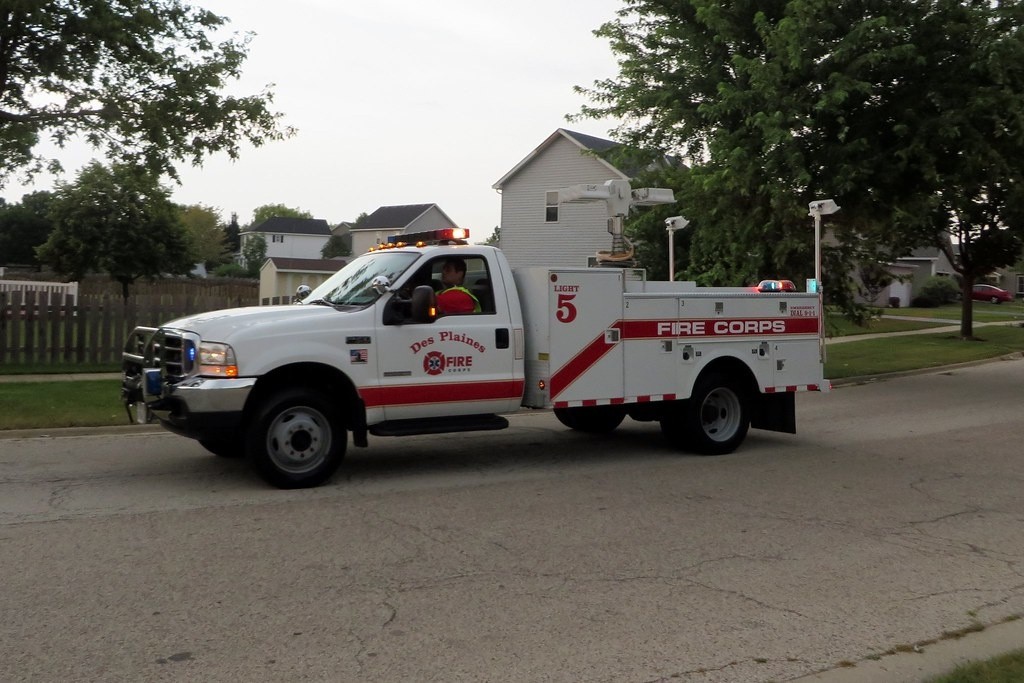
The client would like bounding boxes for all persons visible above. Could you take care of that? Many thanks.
[432,258,481,315]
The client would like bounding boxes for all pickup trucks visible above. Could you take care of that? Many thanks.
[121,180,842,490]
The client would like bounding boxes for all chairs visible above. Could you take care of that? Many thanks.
[471,278,490,296]
[432,278,444,291]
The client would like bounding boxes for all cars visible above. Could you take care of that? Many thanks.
[957,283,1012,305]
